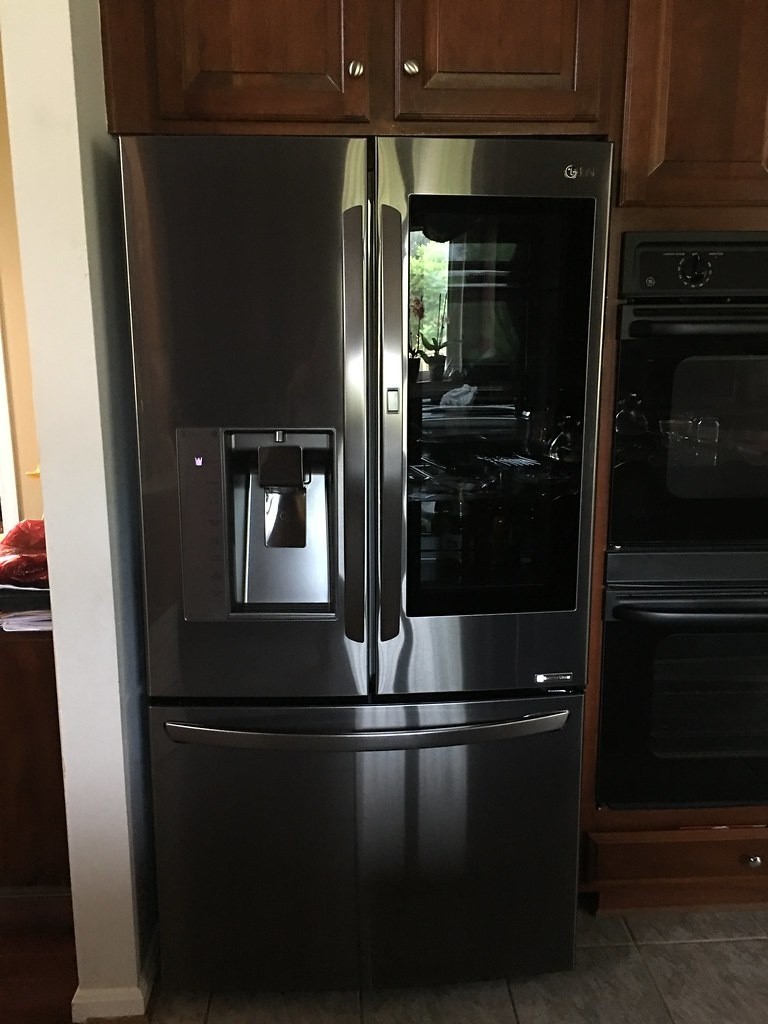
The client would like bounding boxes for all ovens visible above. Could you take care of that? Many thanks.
[593,226,768,810]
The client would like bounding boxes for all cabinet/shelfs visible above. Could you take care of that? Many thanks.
[99,1,611,144]
[579,0,768,916]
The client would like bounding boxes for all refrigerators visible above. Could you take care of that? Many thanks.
[117,132,615,999]
